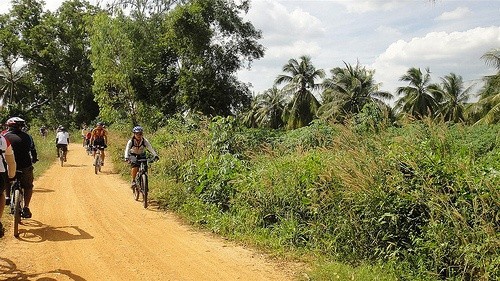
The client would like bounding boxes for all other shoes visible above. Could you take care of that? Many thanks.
[23,207,32,218]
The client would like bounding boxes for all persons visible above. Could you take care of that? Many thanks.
[82,126,89,147]
[55,125,69,162]
[3,117,37,217]
[124,126,159,187]
[0,134,17,232]
[86,128,94,148]
[89,122,108,166]
[40,126,46,136]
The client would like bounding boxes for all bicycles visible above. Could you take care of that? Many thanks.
[124,157,155,208]
[10,169,28,237]
[89,145,105,173]
[58,146,66,166]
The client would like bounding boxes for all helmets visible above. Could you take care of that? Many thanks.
[5,117,26,127]
[132,126,143,133]
[96,122,103,126]
